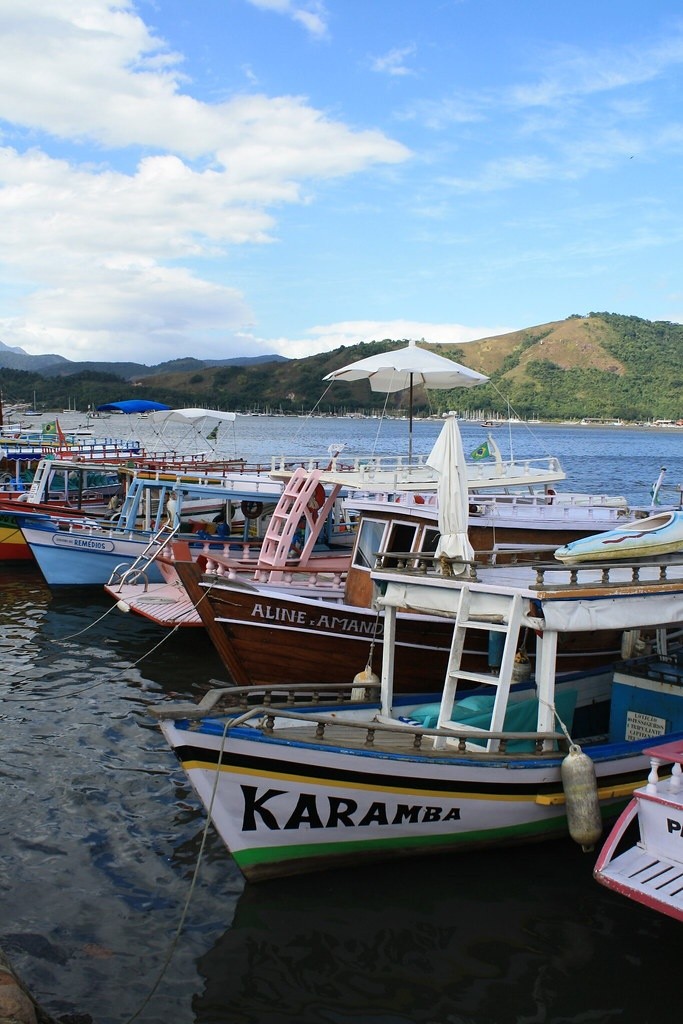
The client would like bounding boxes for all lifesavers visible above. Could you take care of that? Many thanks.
[221,504,236,519]
[240,500,264,519]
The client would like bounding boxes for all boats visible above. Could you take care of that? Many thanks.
[0,405,681,921]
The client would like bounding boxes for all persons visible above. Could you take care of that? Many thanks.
[167,491,181,533]
[288,480,326,558]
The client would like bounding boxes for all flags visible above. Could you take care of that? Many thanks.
[41,420,57,435]
[470,442,490,462]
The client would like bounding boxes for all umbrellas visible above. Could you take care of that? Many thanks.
[426,410,475,578]
[322,339,492,477]
